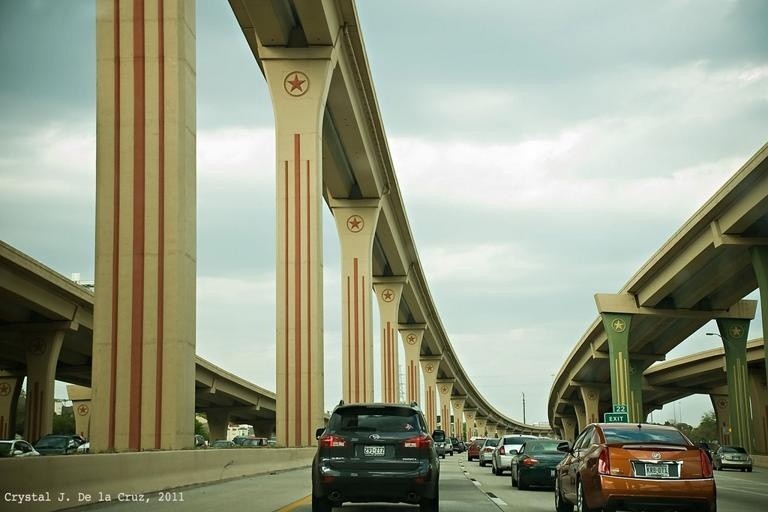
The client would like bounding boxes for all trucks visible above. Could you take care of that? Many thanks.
[241,437,269,447]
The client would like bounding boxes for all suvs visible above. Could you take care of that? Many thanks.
[694,439,719,464]
[311,398,445,510]
[34,434,86,456]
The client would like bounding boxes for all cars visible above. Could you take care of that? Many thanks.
[479,438,502,466]
[77,439,89,454]
[711,444,753,473]
[452,438,462,453]
[211,439,235,447]
[492,434,538,475]
[267,436,276,444]
[0,439,40,457]
[194,434,206,448]
[459,437,473,451]
[443,437,454,458]
[434,440,445,459]
[554,421,717,510]
[468,439,486,461]
[510,439,573,489]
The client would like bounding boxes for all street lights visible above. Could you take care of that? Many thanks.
[706,332,721,341]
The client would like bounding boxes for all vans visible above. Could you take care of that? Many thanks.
[232,435,255,447]
